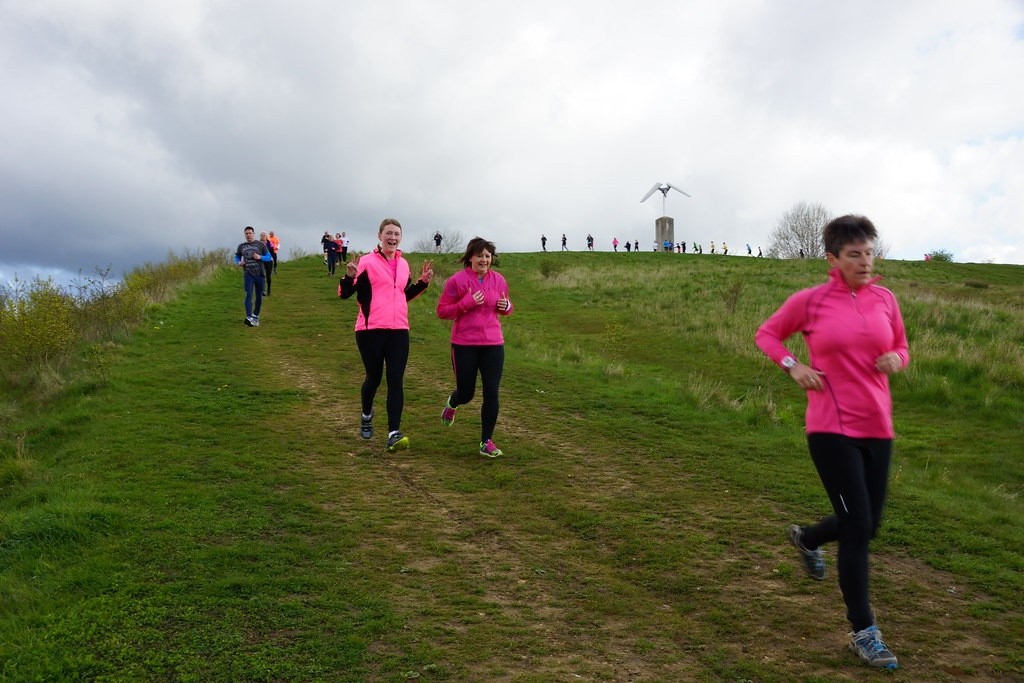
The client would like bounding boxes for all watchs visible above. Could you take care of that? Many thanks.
[782,356,799,372]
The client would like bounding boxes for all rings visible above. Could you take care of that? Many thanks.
[476,296,478,298]
[809,379,814,381]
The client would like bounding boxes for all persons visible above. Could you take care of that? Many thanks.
[561,233,569,251]
[799,249,804,258]
[924,254,930,260]
[587,234,593,250]
[434,231,442,254]
[234,226,271,326]
[746,244,763,257]
[756,215,909,668]
[268,231,279,274]
[260,231,274,296]
[693,241,702,253]
[711,241,728,254]
[652,240,686,253]
[613,238,638,252]
[338,219,434,453]
[321,232,350,276]
[436,238,514,457]
[541,234,547,251]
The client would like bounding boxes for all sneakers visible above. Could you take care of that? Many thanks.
[441,396,458,427]
[479,439,503,459]
[848,624,898,670]
[360,408,373,439]
[323,261,327,265]
[243,313,259,327]
[787,524,828,580]
[262,290,266,296]
[267,293,272,296]
[384,432,410,455]
